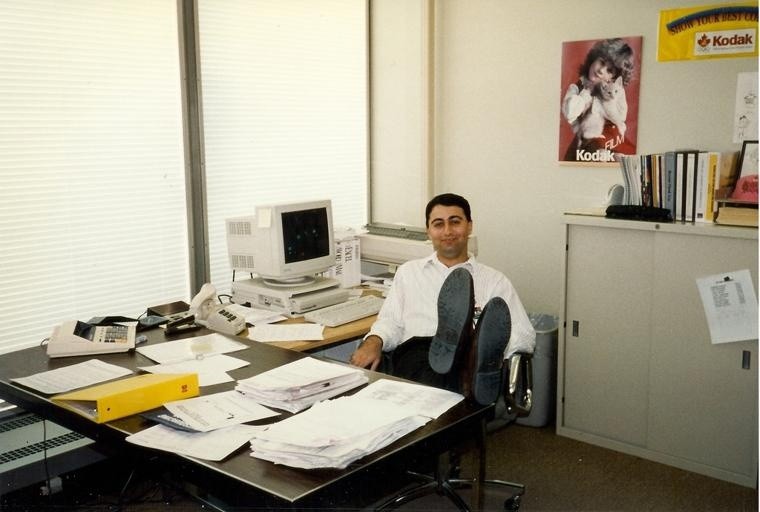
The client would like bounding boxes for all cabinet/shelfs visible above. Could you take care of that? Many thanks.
[557,209,759,490]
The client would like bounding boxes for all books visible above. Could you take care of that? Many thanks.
[234,356,431,469]
[139,410,199,434]
[613,139,758,233]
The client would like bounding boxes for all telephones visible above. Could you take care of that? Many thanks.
[189,283,246,335]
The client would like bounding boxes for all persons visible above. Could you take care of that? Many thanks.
[560,37,635,163]
[350,192,537,410]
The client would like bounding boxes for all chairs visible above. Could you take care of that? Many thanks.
[375,351,536,511]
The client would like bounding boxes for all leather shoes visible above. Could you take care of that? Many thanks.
[427,266,477,374]
[468,296,510,408]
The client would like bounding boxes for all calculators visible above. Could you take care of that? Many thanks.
[46,320,136,358]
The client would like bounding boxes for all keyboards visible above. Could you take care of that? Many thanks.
[303,294,384,328]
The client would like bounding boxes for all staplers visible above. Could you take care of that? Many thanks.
[164,315,202,335]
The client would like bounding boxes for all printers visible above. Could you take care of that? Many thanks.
[356,222,478,281]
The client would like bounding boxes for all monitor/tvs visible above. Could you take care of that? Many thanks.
[224,199,335,288]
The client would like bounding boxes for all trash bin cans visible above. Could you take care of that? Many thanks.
[515,311,558,427]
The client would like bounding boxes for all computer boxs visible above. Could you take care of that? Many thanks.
[231,275,349,318]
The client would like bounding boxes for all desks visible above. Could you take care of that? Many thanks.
[0,280,497,512]
